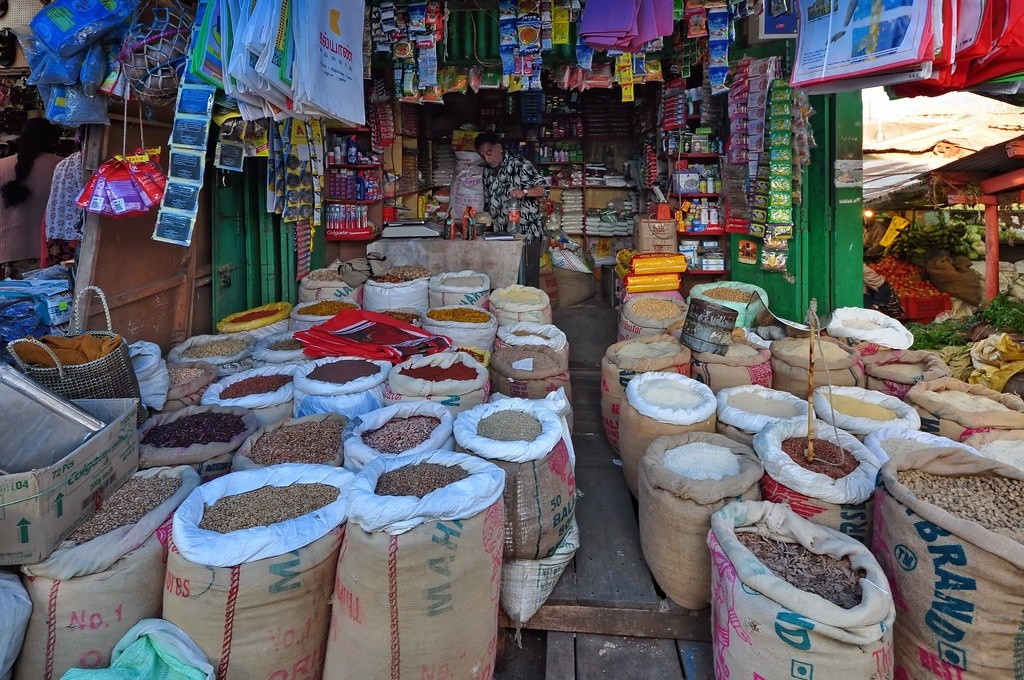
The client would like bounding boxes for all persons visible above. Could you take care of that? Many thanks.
[0,118,65,280]
[474,130,550,289]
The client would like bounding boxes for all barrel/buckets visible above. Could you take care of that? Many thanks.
[680,298,739,355]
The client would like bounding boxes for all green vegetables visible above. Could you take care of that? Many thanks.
[902,292,1024,351]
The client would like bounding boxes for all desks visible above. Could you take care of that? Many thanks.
[367,234,523,290]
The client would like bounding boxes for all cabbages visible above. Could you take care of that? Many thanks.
[952,225,1016,261]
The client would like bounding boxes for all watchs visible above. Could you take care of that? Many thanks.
[522,188,528,198]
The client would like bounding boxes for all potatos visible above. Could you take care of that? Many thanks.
[123,40,190,89]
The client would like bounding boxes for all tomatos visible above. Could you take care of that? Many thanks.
[869,255,942,297]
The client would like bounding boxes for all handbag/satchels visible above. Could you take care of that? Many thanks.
[291,305,453,362]
[7,285,148,430]
[16,0,367,127]
[789,0,1024,101]
[45,150,85,240]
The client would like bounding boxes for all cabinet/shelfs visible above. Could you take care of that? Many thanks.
[325,89,732,274]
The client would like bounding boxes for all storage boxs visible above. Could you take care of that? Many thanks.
[601,264,624,308]
[452,129,485,151]
[634,217,679,254]
[0,398,141,567]
[672,171,700,194]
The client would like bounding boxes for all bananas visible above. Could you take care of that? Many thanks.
[890,223,968,267]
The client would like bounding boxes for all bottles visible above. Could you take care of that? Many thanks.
[710,209,717,224]
[507,199,519,233]
[553,143,584,163]
[699,175,722,193]
[701,209,708,224]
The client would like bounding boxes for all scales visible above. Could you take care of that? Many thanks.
[381,217,445,240]
